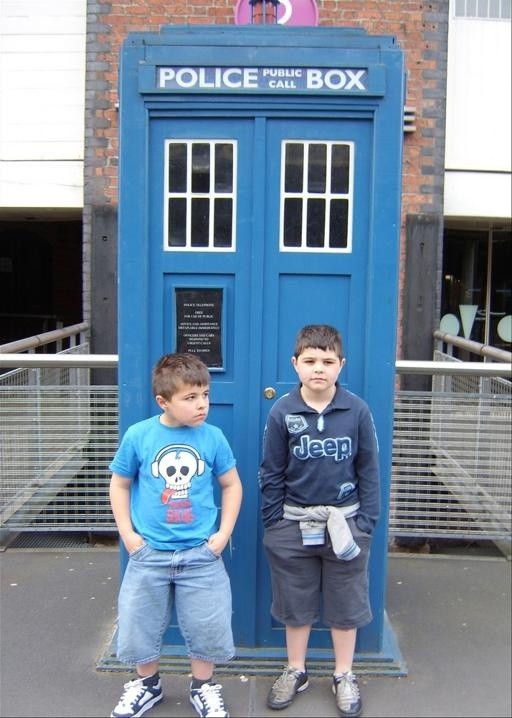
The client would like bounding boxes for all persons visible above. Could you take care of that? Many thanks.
[257,323,380,718]
[108,353,243,718]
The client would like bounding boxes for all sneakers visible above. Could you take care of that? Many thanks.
[267,663,309,709]
[110,674,164,718]
[189,679,229,718]
[331,671,362,718]
